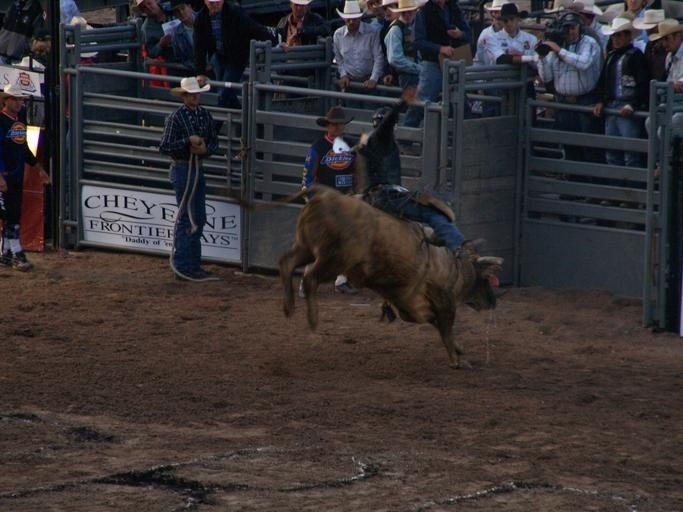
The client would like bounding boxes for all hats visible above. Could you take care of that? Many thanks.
[316,106,353,126]
[65,15,98,58]
[0,85,30,103]
[132,0,202,12]
[335,0,422,19]
[544,1,683,42]
[484,0,528,20]
[172,77,210,97]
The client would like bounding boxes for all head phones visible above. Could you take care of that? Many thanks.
[559,13,586,34]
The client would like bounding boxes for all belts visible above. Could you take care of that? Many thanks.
[171,159,201,168]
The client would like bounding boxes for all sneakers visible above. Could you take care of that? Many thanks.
[0,249,32,272]
[335,282,358,295]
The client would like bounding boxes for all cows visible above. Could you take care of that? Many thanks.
[227,183,505,369]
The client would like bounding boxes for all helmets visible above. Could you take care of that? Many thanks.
[371,107,398,129]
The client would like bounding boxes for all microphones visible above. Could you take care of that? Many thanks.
[572,34,585,44]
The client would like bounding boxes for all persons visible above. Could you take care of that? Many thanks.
[295,106,357,298]
[0,84,51,272]
[1,2,682,232]
[159,77,214,282]
[354,85,487,258]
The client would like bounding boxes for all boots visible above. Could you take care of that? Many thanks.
[395,105,424,156]
